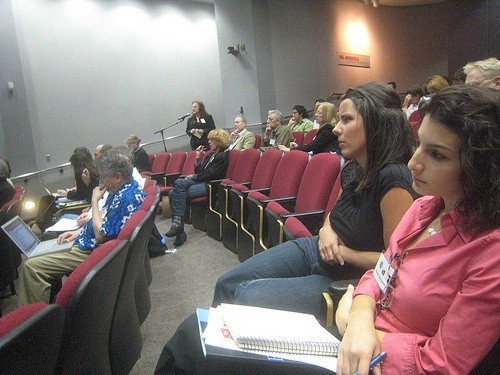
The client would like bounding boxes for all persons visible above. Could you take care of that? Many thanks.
[259,110,294,152]
[154,85,500,375]
[186,101,216,151]
[212,80,417,320]
[224,115,256,152]
[287,105,314,132]
[314,99,327,129]
[165,129,231,246]
[386,57,500,120]
[278,102,341,155]
[19,135,149,307]
[0,159,16,210]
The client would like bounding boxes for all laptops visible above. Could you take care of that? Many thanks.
[42,184,68,198]
[1,215,76,259]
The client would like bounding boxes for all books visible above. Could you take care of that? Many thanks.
[196,302,343,373]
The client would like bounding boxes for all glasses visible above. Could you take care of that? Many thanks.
[341,87,364,101]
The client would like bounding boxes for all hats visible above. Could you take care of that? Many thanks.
[124,134,142,143]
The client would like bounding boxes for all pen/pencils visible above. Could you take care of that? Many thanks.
[354,351,387,375]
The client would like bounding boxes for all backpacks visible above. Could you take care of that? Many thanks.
[147,224,167,259]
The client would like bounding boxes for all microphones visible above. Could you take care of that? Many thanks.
[178,112,190,120]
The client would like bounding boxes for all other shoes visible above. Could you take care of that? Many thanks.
[173,232,187,247]
[165,222,184,237]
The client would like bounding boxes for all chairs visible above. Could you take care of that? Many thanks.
[303,129,318,145]
[408,110,423,143]
[0,185,26,296]
[254,136,261,149]
[0,302,65,375]
[54,239,129,375]
[107,148,347,375]
[292,132,305,144]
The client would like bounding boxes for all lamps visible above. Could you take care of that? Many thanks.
[228,43,246,53]
[365,0,379,7]
[8,82,13,90]
